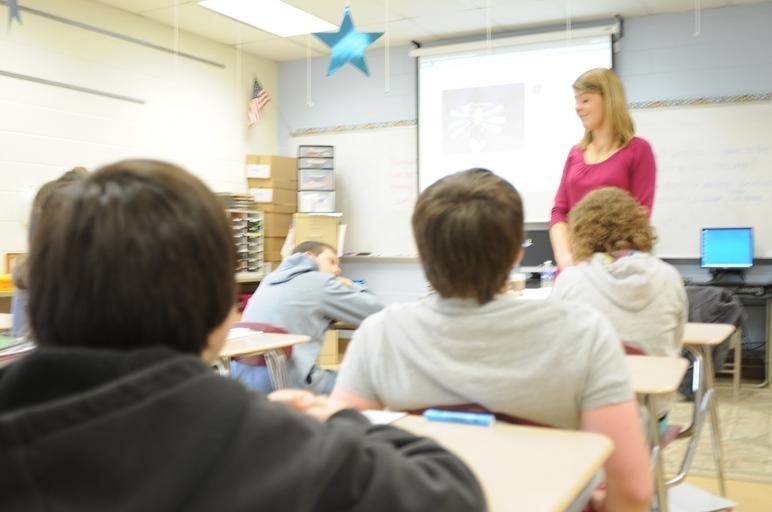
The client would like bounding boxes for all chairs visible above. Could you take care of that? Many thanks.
[679,286,742,401]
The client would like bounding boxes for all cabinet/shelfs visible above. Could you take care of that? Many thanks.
[292,212,343,266]
[224,210,264,271]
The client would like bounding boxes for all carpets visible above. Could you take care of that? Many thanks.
[662,378,772,485]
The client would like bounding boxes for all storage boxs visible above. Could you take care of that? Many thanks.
[298,158,334,169]
[298,144,334,158]
[298,170,335,191]
[263,261,282,276]
[297,191,335,213]
[247,187,299,213]
[254,210,293,237]
[263,237,287,261]
[247,153,298,190]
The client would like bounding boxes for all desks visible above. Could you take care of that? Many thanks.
[623,354,690,512]
[682,281,772,388]
[218,327,310,390]
[683,322,737,499]
[317,322,359,372]
[267,389,616,512]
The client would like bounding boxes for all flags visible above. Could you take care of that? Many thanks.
[247,78,271,130]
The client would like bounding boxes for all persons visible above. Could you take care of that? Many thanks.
[329,165,658,512]
[228,239,387,401]
[2,157,487,512]
[551,184,689,446]
[9,253,29,340]
[547,67,657,278]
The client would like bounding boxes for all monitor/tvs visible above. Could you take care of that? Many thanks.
[701,227,753,286]
[517,230,558,289]
